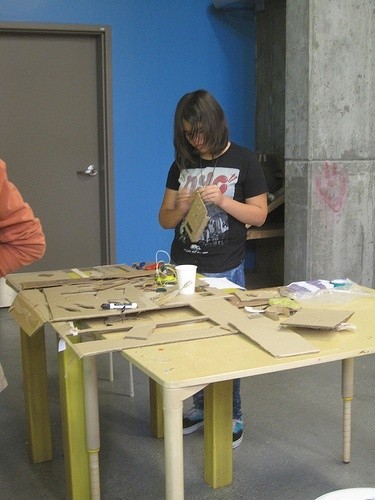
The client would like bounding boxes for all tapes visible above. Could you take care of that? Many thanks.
[330,278,347,287]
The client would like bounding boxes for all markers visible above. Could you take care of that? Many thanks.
[101,302,139,310]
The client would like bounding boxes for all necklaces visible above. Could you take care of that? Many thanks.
[199,153,216,188]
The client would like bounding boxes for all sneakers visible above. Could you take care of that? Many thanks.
[232,414,244,448]
[183,407,204,435]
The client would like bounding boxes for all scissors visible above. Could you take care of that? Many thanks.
[131,261,145,270]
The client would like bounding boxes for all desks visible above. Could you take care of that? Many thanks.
[5,269,375,500]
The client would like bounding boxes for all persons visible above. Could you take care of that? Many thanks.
[159,89,268,448]
[0,158,46,278]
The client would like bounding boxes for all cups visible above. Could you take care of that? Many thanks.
[175,265,198,294]
